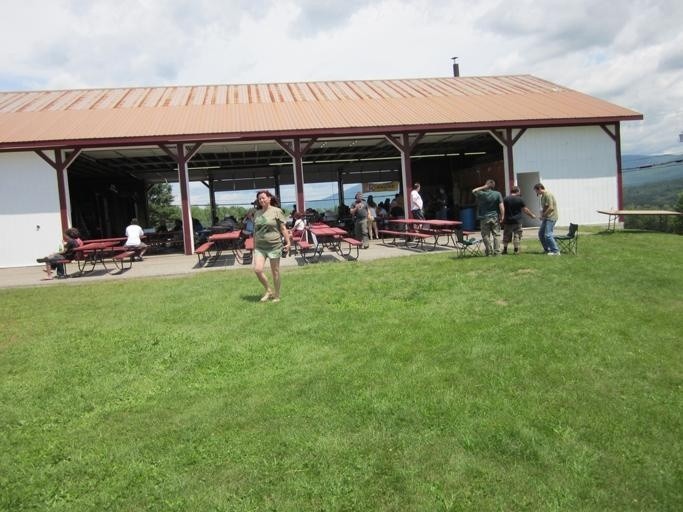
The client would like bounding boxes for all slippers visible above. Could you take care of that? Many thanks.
[261,295,272,302]
[273,298,280,302]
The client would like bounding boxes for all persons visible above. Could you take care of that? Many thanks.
[236,207,254,247]
[534,183,560,256]
[125,218,149,261]
[43,227,81,274]
[342,190,405,249]
[436,187,448,219]
[284,212,304,247]
[156,221,169,248]
[192,217,204,244]
[292,204,296,228]
[57,228,83,277]
[306,208,323,222]
[501,185,536,254]
[173,221,184,246]
[249,191,291,302]
[411,183,425,232]
[471,180,505,255]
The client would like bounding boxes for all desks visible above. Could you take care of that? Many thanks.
[83,234,147,262]
[597,208,682,234]
[208,229,243,267]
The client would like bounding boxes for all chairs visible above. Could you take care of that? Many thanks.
[454,226,483,258]
[553,223,579,256]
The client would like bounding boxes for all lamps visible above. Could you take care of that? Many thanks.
[172,165,222,171]
[268,152,488,166]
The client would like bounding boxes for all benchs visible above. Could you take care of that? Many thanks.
[37,242,140,279]
[194,238,254,267]
[377,218,470,253]
[289,222,363,265]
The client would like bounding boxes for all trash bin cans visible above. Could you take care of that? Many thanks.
[458,206,474,231]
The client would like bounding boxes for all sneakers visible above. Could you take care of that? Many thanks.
[548,252,560,256]
